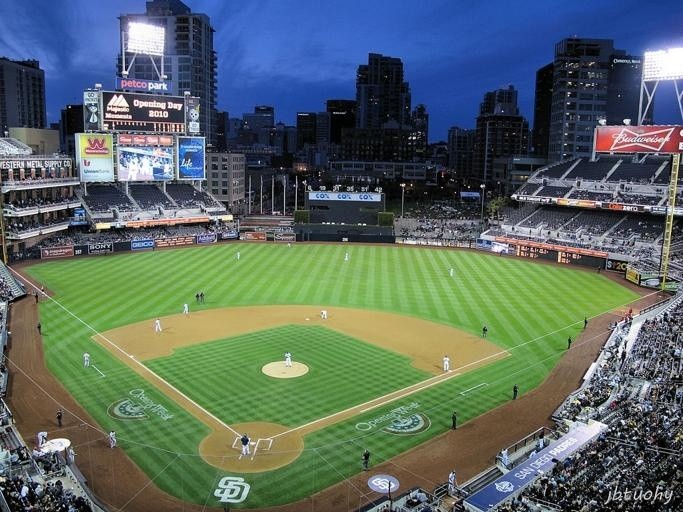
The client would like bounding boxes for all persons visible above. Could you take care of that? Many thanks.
[199,292,204,303]
[343,251,349,264]
[483,299,682,510]
[399,174,682,283]
[0,175,234,265]
[121,154,170,180]
[155,318,161,333]
[451,410,459,430]
[195,293,198,303]
[183,303,188,315]
[286,242,290,247]
[443,355,450,370]
[108,429,116,449]
[447,470,457,493]
[321,311,327,319]
[0,276,91,511]
[236,250,240,260]
[362,448,369,469]
[284,351,292,368]
[240,433,250,456]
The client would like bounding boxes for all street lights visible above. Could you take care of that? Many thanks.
[496,181,500,198]
[399,182,406,219]
[478,182,485,225]
[422,177,471,205]
[301,178,307,209]
[232,180,239,213]
